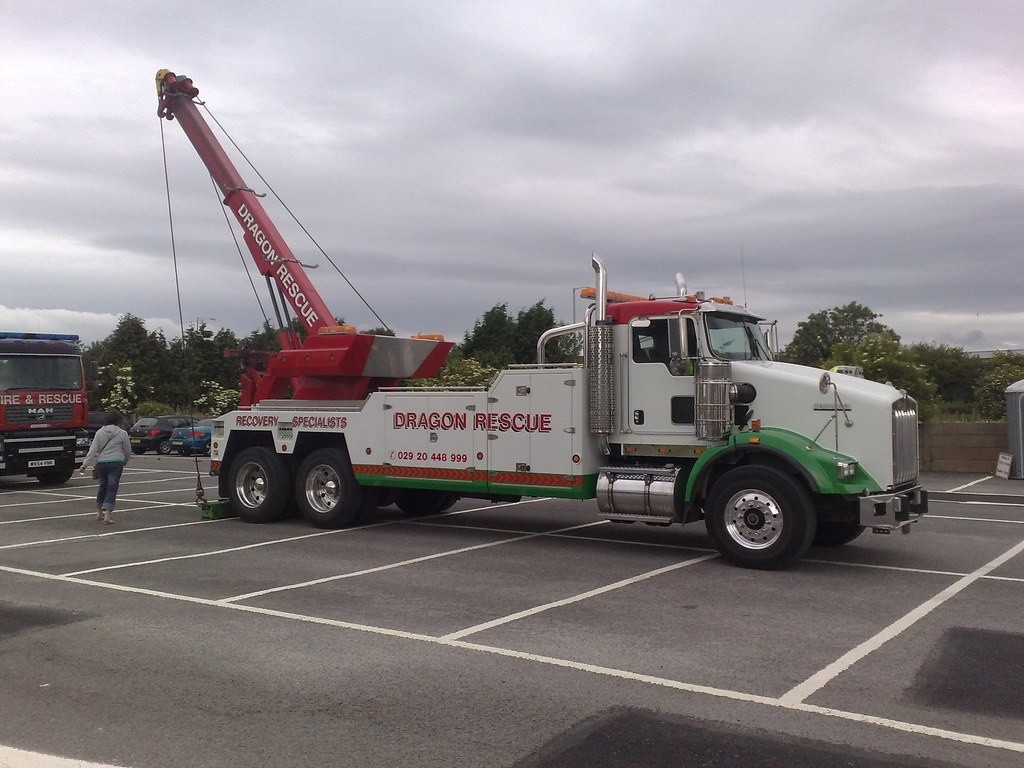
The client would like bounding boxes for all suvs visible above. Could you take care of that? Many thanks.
[129,415,200,456]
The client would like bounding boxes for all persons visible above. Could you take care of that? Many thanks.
[79,409,132,525]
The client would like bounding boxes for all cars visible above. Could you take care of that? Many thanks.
[169,419,217,457]
[76,426,88,458]
[85,412,136,457]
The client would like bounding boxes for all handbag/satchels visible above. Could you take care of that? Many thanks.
[92,463,99,481]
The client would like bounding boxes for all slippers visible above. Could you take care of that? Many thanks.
[105,520,114,525]
[97,515,105,520]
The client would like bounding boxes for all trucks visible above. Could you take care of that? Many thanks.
[0,332,88,486]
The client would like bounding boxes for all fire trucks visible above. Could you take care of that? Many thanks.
[154,65,933,570]
